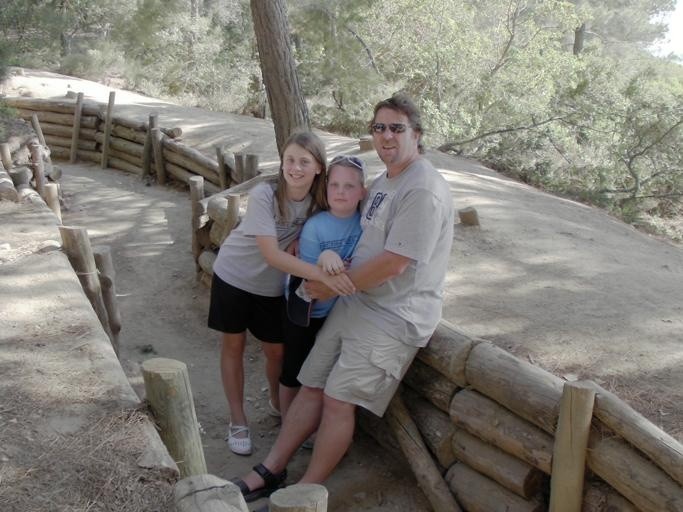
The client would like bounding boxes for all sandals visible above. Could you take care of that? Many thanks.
[226,463,286,502]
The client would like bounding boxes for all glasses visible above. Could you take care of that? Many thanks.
[372,122,406,133]
[330,155,364,168]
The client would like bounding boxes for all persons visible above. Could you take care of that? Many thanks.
[208,133,356,456]
[279,155,366,449]
[228,95,455,502]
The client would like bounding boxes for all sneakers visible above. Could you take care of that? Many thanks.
[228,420,251,455]
